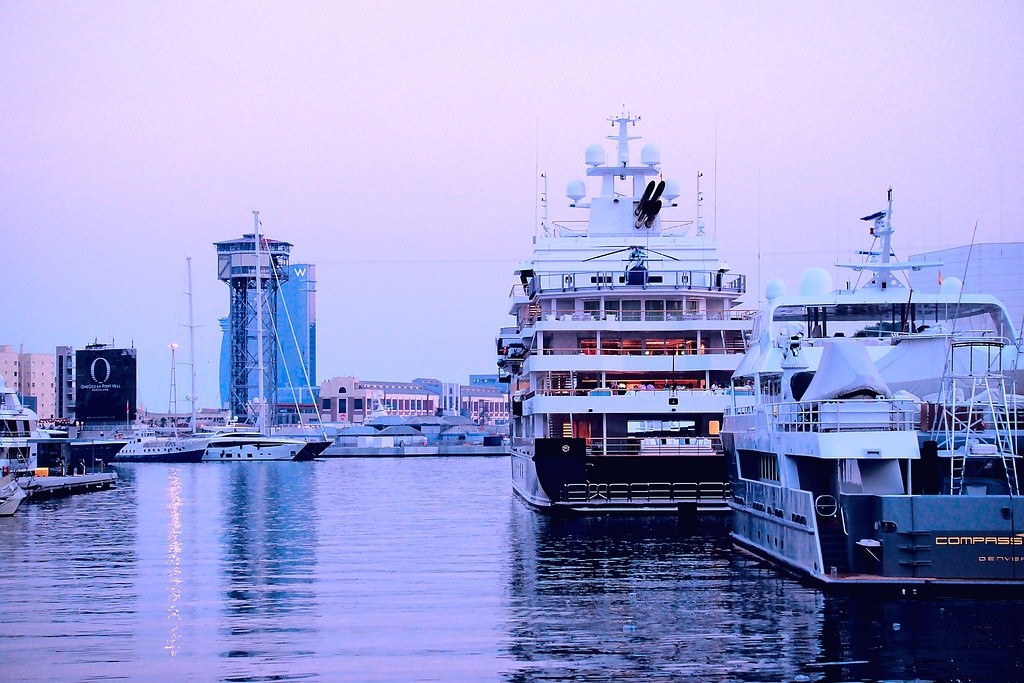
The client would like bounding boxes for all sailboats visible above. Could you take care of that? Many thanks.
[202,210,335,462]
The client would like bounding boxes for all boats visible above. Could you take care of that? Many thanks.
[111,435,211,464]
[715,185,1024,597]
[494,101,764,533]
[0,372,129,498]
